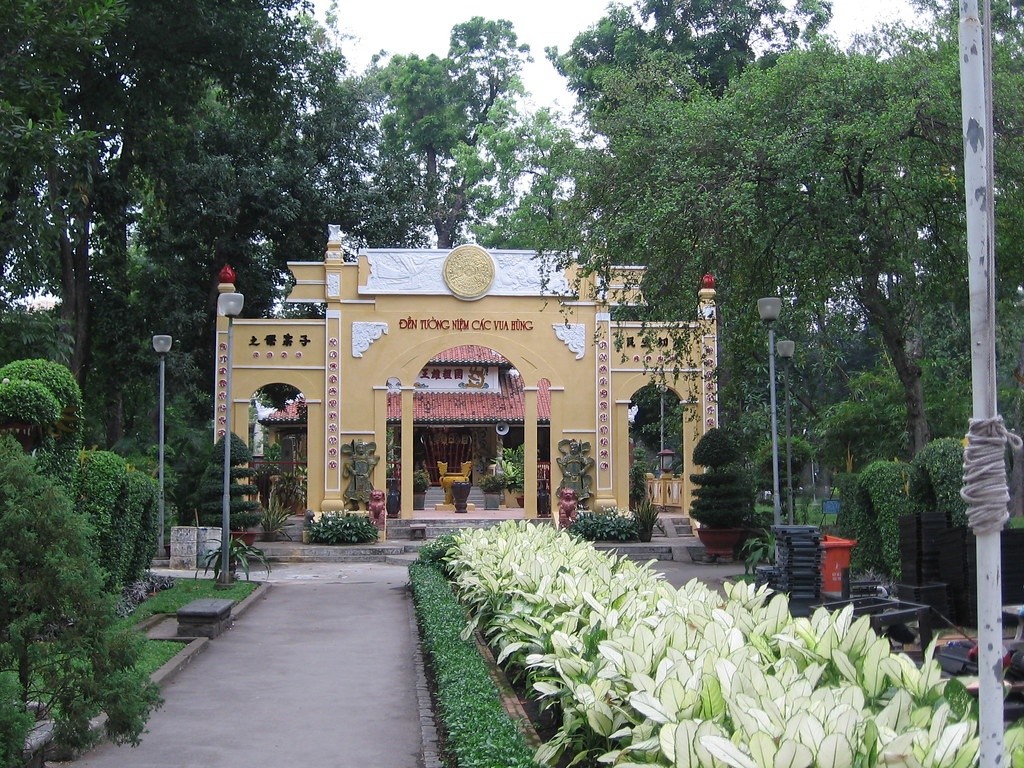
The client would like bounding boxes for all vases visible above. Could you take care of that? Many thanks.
[386,477,401,518]
[537,479,550,518]
[451,481,471,513]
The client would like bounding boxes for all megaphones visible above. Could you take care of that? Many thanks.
[496,421,509,436]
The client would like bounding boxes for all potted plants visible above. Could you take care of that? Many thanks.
[479,474,507,511]
[251,464,308,515]
[414,469,432,510]
[258,498,296,542]
[689,426,757,556]
[194,532,272,583]
[306,512,381,546]
[514,443,541,508]
[230,484,264,546]
[629,498,660,543]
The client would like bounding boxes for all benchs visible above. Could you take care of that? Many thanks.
[176,598,236,640]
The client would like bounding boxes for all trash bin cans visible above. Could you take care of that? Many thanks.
[817,534,857,600]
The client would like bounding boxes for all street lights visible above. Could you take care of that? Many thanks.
[756,295,784,528]
[776,339,798,528]
[152,335,175,560]
[214,292,244,591]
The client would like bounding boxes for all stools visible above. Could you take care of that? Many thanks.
[410,523,428,539]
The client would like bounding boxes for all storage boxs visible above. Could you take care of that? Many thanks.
[754,510,978,631]
